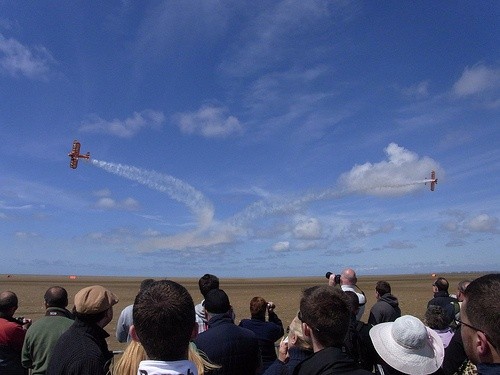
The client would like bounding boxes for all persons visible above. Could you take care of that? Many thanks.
[0,269,500,375]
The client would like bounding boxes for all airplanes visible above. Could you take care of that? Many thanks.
[68,140,91,169]
[424,171,438,191]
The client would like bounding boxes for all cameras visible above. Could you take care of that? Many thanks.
[325,272,341,284]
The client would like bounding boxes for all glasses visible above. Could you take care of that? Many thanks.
[287,325,302,334]
[297,310,320,332]
[454,315,496,348]
[432,283,436,287]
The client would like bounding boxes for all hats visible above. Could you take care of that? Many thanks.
[202,289,231,314]
[74,285,119,314]
[369,315,444,375]
